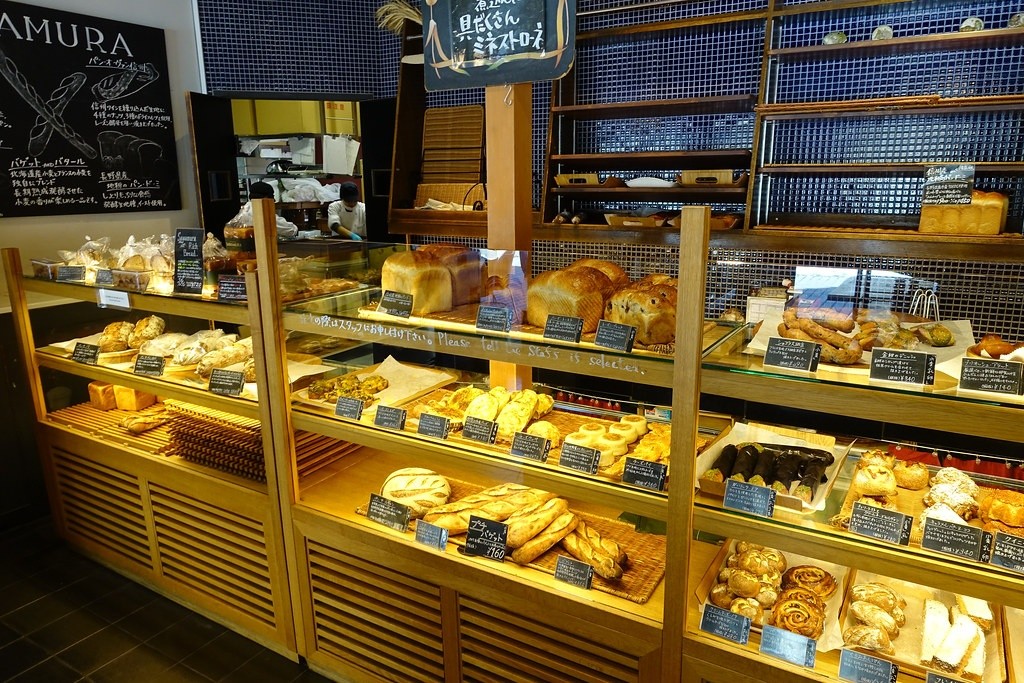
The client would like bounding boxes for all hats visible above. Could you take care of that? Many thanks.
[249,181,274,194]
[340,182,358,203]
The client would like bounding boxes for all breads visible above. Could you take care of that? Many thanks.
[526,258,679,346]
[701,441,834,505]
[380,468,627,581]
[852,448,1024,548]
[309,374,388,409]
[381,242,482,316]
[412,383,671,478]
[918,189,1009,234]
[87,380,169,433]
[96,315,256,382]
[74,224,359,302]
[711,540,995,681]
[776,303,1024,366]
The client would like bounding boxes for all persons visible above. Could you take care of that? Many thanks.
[244,181,298,239]
[328,181,365,242]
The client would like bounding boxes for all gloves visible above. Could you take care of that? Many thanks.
[350,233,362,240]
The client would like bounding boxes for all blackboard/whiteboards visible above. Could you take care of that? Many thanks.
[420,0,576,93]
[0,0,185,221]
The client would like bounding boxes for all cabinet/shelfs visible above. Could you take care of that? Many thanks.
[234,134,362,237]
[382,0,1024,234]
[0,203,1024,683]
